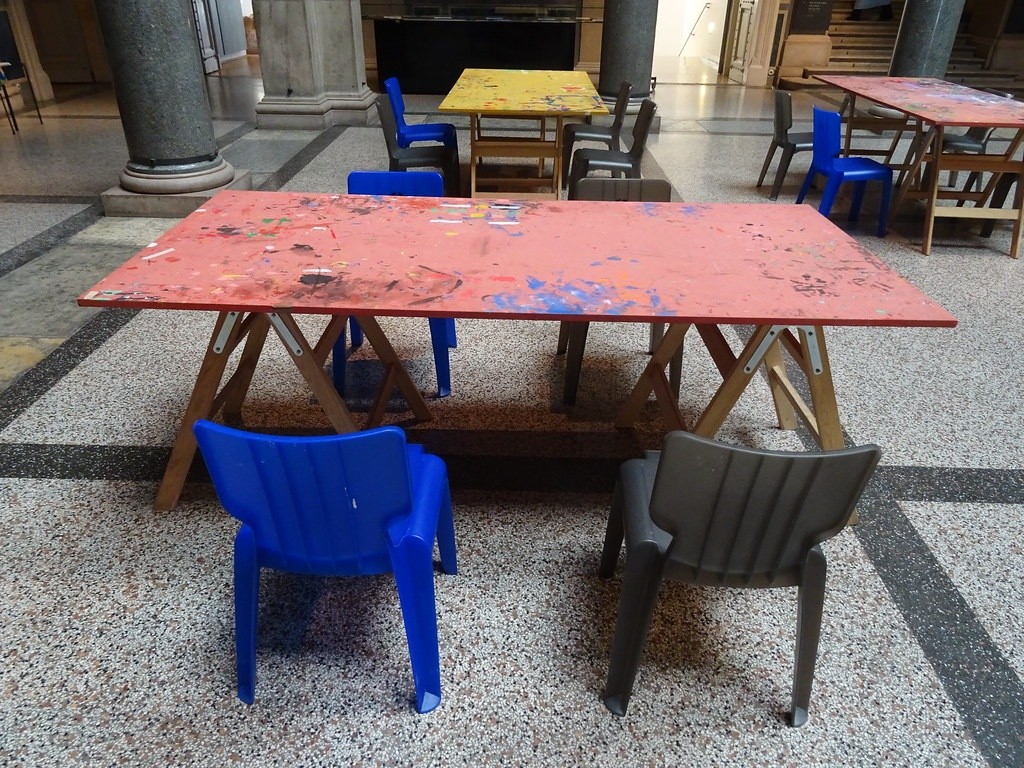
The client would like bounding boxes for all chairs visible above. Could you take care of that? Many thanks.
[561,81,631,186]
[385,76,457,146]
[796,103,892,237]
[568,98,659,199]
[373,95,461,197]
[914,86,1013,201]
[756,89,813,201]
[330,169,458,396]
[554,176,683,403]
[189,417,459,713]
[957,170,1018,237]
[599,431,883,726]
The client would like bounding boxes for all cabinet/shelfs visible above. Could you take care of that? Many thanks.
[362,15,605,95]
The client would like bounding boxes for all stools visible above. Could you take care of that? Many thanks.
[0,61,43,135]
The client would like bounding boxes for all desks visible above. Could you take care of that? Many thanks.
[437,68,610,200]
[77,188,957,524]
[812,74,1024,259]
